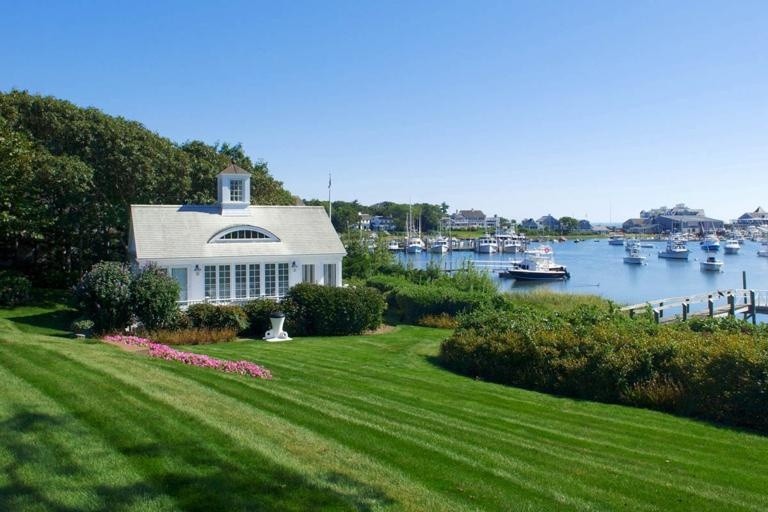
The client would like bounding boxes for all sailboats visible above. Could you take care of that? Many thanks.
[344,204,567,254]
[574,213,768,271]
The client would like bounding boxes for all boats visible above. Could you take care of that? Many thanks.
[506,231,570,280]
